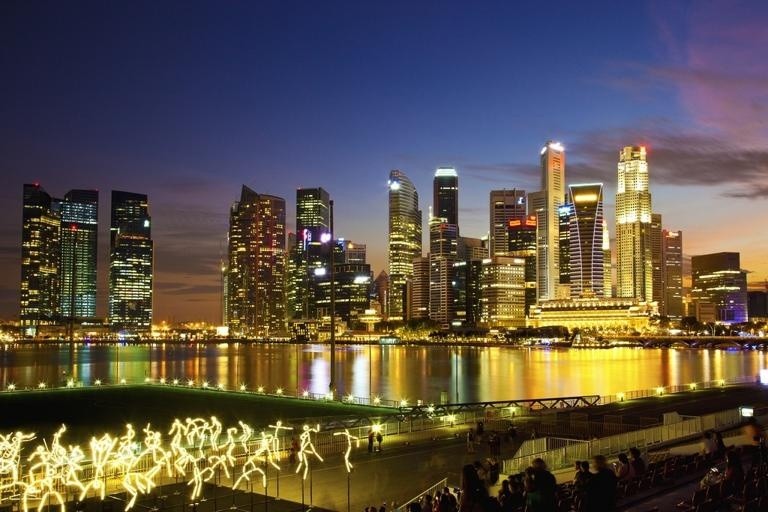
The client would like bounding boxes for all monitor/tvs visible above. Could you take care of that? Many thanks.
[741,408,754,417]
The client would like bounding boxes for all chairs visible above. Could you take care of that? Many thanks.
[445,443,768,512]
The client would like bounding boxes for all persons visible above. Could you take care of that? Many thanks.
[288,448,297,472]
[699,417,767,511]
[368,429,383,453]
[466,419,519,456]
[406,457,503,511]
[291,437,298,449]
[498,448,645,510]
[379,502,387,511]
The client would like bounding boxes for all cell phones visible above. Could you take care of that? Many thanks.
[613,463,617,467]
[710,467,719,473]
